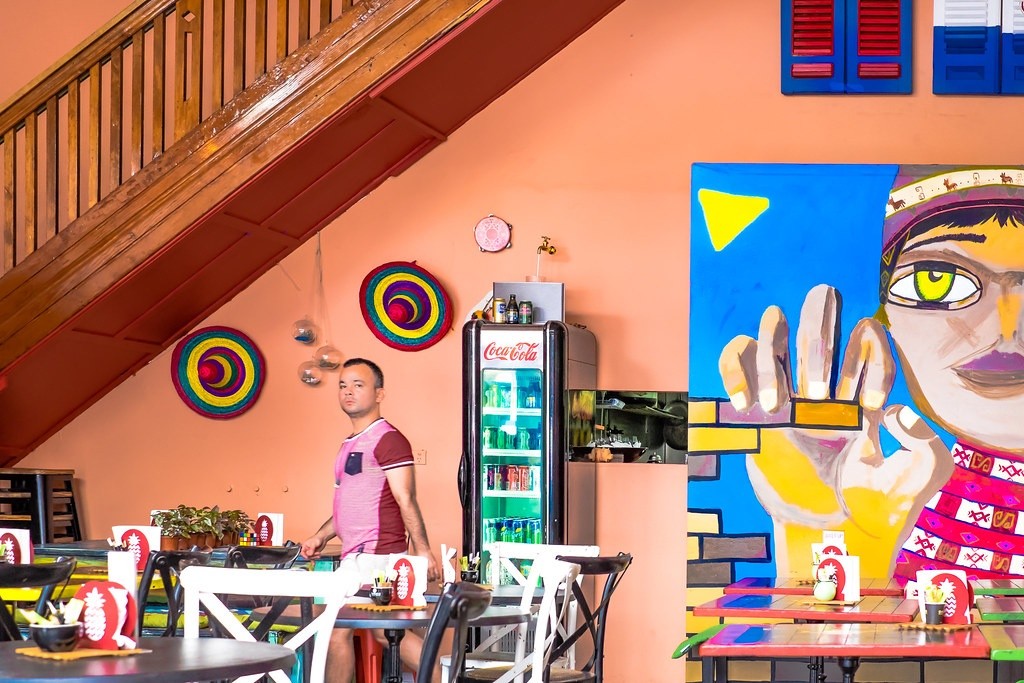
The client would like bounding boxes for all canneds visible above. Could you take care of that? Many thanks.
[518,300,533,324]
[485,517,542,545]
[493,298,506,324]
[484,385,541,491]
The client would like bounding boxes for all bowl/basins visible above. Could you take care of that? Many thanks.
[461,570,478,584]
[29,621,84,652]
[369,586,393,606]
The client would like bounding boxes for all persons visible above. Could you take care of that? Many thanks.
[300,358,442,683]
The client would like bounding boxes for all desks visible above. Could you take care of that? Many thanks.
[250,602,533,682]
[694,576,1024,683]
[421,582,577,683]
[0,636,295,683]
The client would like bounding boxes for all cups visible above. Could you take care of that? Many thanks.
[813,580,836,601]
[925,603,945,625]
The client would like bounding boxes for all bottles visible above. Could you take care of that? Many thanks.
[506,294,519,324]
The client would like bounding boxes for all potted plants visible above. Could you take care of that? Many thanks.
[151,503,255,553]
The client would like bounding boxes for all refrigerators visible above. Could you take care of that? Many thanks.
[458,319,597,672]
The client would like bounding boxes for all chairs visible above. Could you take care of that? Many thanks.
[417,541,633,682]
[135,544,366,683]
[0,555,79,641]
